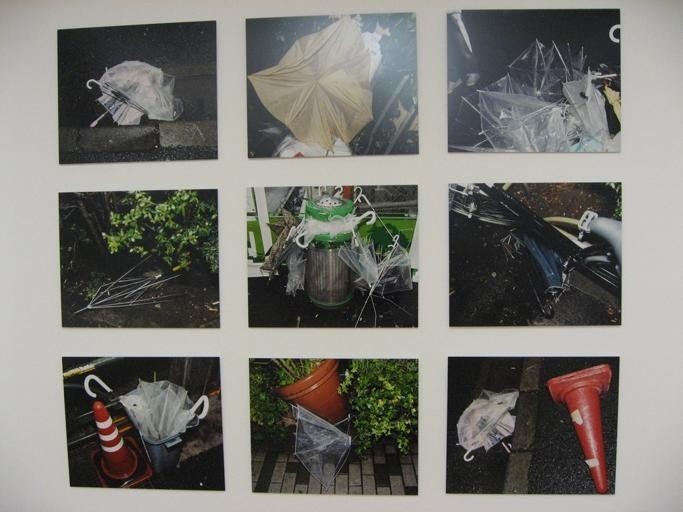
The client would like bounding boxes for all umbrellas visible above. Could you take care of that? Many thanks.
[457,387,520,462]
[84,374,210,443]
[524,270,564,320]
[259,186,414,296]
[87,79,150,114]
[449,24,621,152]
[288,401,353,493]
[247,16,375,152]
[85,60,184,130]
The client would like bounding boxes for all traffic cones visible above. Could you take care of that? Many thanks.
[88,401,153,489]
[546,362,614,492]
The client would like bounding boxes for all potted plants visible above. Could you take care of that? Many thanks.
[270,358,348,427]
